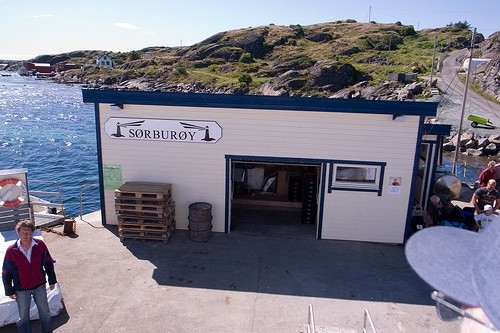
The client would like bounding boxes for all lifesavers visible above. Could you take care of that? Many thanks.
[0,178,26,207]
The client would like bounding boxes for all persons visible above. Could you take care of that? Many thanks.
[1,219,57,333]
[474,204,500,232]
[423,194,475,227]
[403,220,500,333]
[471,178,500,232]
[478,160,498,188]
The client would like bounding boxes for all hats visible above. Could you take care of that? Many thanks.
[482,204,492,211]
